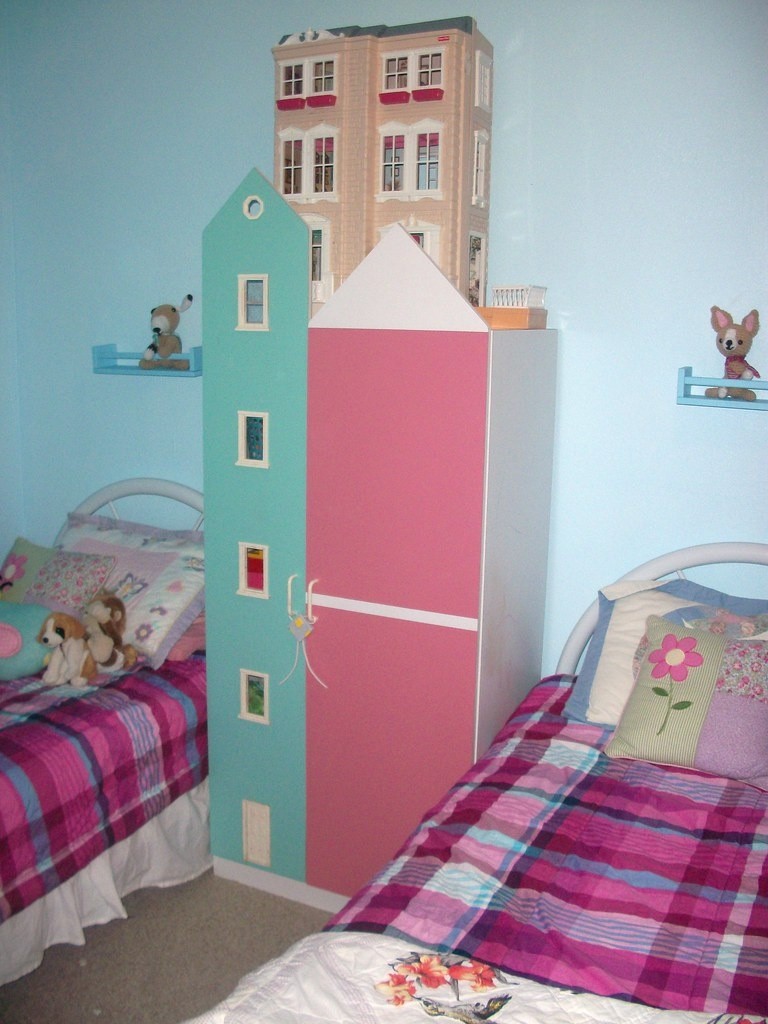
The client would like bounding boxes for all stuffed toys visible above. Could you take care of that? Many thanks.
[705,305,760,401]
[138,294,193,371]
[37,612,97,687]
[82,594,137,670]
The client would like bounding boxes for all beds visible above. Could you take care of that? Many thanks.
[179,541,768,1024]
[0,474,206,986]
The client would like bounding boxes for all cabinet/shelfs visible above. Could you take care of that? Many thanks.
[285,147,332,193]
[199,166,554,915]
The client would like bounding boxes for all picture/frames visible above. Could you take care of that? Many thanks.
[391,168,399,177]
[391,156,399,166]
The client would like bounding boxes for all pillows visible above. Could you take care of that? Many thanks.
[53,512,205,670]
[0,536,119,619]
[558,577,768,732]
[602,614,768,794]
